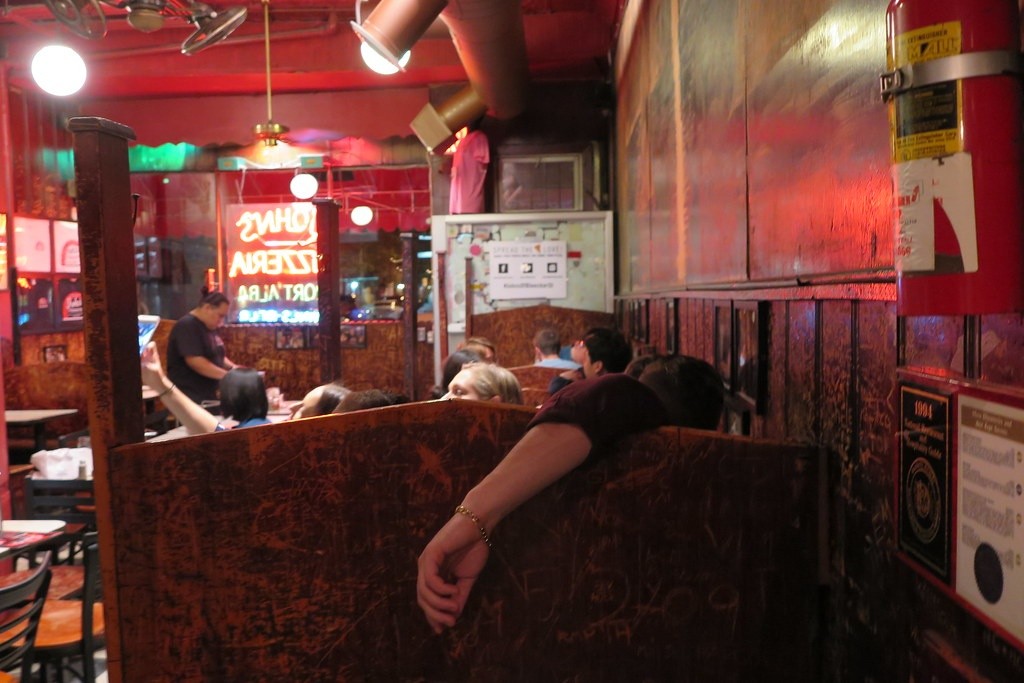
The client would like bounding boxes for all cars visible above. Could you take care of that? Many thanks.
[339,291,358,321]
[373,299,404,314]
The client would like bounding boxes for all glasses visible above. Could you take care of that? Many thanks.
[656,352,679,400]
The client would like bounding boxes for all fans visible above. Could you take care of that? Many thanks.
[168,0,248,55]
[43,0,119,40]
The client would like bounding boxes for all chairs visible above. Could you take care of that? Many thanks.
[0,477,107,683]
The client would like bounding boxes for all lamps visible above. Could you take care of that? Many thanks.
[126,8,165,34]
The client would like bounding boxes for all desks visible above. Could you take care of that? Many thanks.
[4,409,78,450]
[0,518,87,565]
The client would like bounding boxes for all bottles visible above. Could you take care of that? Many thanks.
[79,460,87,480]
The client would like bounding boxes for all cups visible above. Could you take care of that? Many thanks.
[265,387,279,410]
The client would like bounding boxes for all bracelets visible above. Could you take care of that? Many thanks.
[158,384,174,398]
[453,506,493,548]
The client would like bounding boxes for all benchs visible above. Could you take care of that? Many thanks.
[5,362,94,451]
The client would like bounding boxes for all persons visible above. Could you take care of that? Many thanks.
[219,367,274,430]
[167,286,266,415]
[136,275,151,315]
[425,337,524,408]
[315,293,357,384]
[533,328,634,404]
[141,341,391,436]
[416,353,725,633]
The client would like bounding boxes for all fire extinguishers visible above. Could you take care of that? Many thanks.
[882,0,1024,318]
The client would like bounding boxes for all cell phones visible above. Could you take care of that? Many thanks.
[137,314,160,355]
